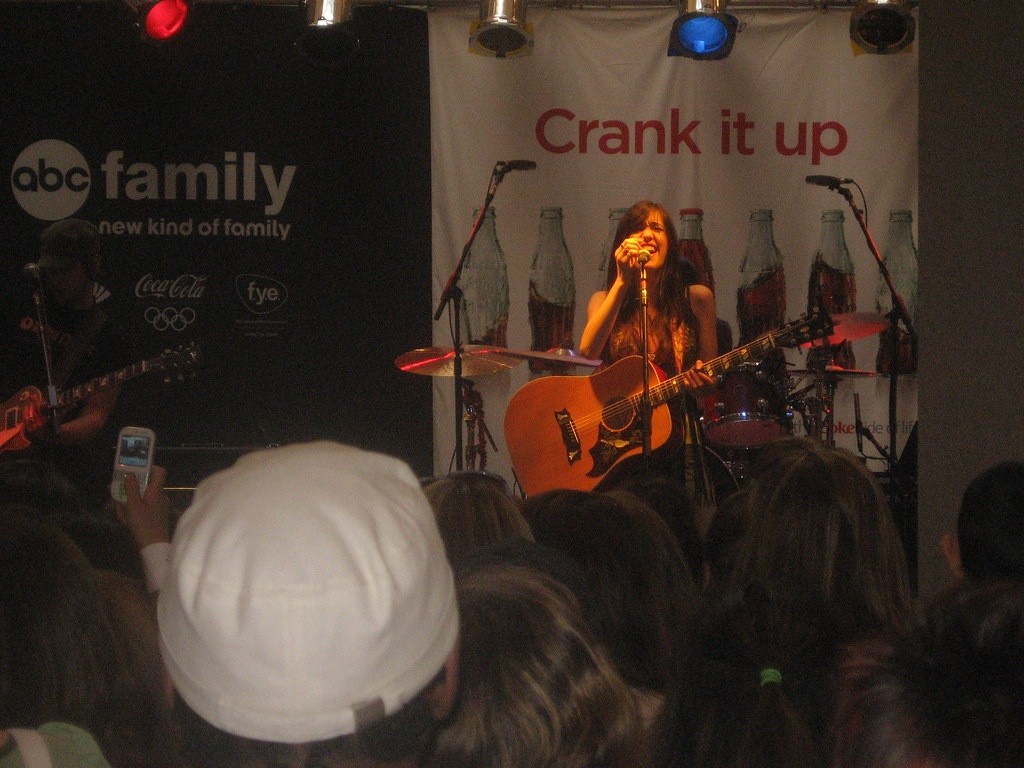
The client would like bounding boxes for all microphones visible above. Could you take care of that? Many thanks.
[805,175,854,186]
[853,393,863,452]
[497,160,537,171]
[23,263,42,284]
[637,250,651,267]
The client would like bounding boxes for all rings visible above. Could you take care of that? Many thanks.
[621,242,625,248]
[624,246,629,252]
[626,253,632,257]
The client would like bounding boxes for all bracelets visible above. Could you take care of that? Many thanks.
[138,541,169,590]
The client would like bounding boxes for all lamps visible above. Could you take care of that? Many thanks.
[293,0,367,71]
[848,0,916,55]
[667,0,740,61]
[468,0,534,60]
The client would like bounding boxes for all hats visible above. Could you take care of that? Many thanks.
[155,440,460,744]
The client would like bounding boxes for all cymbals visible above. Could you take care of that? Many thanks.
[788,365,883,378]
[490,345,603,368]
[800,310,891,349]
[394,345,525,378]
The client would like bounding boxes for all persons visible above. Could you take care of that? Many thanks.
[0,436,1023,768]
[579,201,719,401]
[0,218,137,465]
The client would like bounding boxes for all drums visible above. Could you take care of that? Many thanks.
[698,360,794,450]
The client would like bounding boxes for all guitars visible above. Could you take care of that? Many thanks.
[0,341,205,454]
[502,305,836,499]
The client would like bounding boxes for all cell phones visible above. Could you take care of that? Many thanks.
[110,426,156,504]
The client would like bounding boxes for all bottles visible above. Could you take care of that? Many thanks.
[877,210,919,375]
[596,208,630,296]
[463,208,508,349]
[734,207,785,367]
[807,211,856,369]
[527,207,575,372]
[678,209,714,296]
